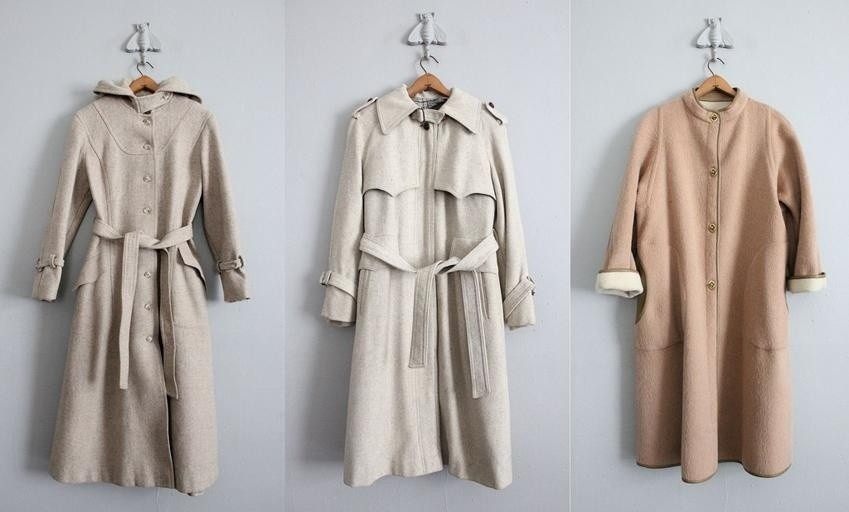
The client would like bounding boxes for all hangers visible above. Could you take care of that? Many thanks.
[694,56,737,102]
[404,55,451,104]
[126,59,160,97]
